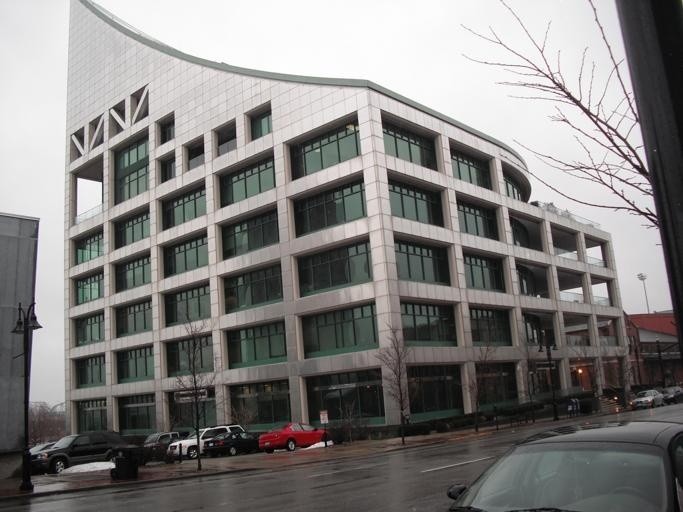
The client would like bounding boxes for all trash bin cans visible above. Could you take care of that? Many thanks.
[110,445,140,480]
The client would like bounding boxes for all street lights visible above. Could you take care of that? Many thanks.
[12,302,42,491]
[538,329,560,421]
[638,274,649,313]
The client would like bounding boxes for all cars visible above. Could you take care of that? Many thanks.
[447,421,683,512]
[632,385,683,409]
